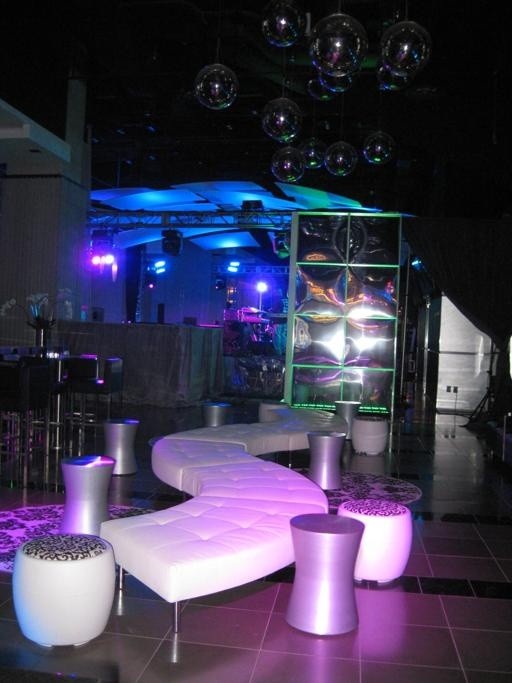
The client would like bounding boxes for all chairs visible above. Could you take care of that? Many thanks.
[0,346,124,464]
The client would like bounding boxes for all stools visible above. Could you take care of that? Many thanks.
[58,453,116,535]
[102,418,140,475]
[337,498,413,584]
[283,513,361,638]
[351,415,389,456]
[335,402,362,441]
[102,462,331,634]
[152,403,350,492]
[13,533,118,649]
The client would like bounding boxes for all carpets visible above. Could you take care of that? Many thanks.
[0,504,157,572]
[293,468,422,510]
